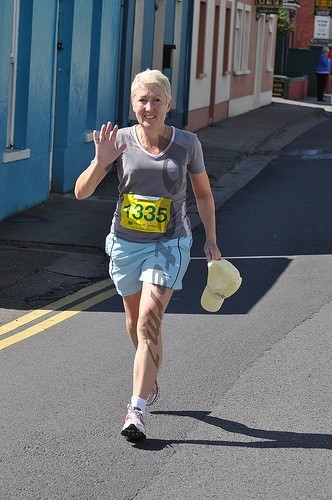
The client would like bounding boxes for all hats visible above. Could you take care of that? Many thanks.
[201,259,242,312]
[322,47,329,52]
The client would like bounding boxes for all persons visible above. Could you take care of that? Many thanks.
[316,46,332,103]
[75,68,222,444]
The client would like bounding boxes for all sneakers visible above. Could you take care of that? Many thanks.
[117,404,147,445]
[142,380,160,406]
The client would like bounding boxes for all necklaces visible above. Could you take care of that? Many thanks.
[139,125,169,156]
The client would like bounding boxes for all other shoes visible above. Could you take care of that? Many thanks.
[318,99,326,103]
